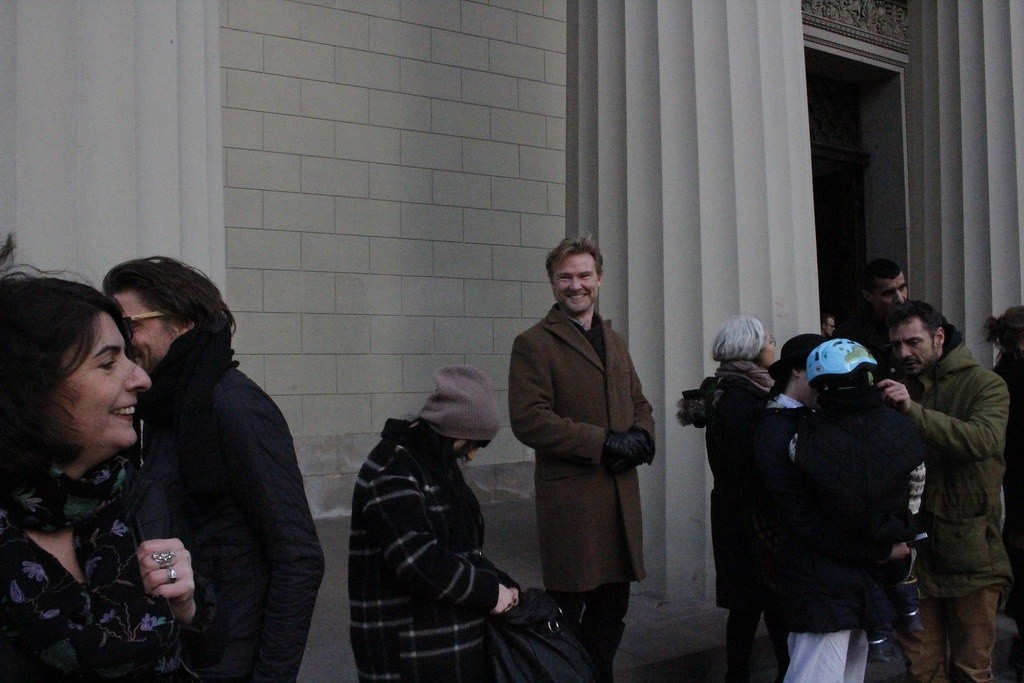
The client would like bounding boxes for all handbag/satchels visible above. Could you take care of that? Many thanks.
[486,587,603,683]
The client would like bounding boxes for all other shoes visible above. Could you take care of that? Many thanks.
[893,610,924,633]
[867,637,904,663]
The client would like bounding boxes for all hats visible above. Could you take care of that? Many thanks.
[417,365,501,441]
[768,334,827,380]
[712,318,763,360]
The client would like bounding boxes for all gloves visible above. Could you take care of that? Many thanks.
[603,427,654,475]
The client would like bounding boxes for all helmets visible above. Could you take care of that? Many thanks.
[806,338,878,390]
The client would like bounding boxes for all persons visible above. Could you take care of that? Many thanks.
[508,235,658,683]
[0,230,215,683]
[679,256,1024,683]
[102,256,326,683]
[348,365,525,683]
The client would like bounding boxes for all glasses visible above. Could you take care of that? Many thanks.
[764,338,776,345]
[124,309,165,334]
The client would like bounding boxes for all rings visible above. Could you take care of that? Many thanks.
[151,551,175,564]
[168,567,176,583]
